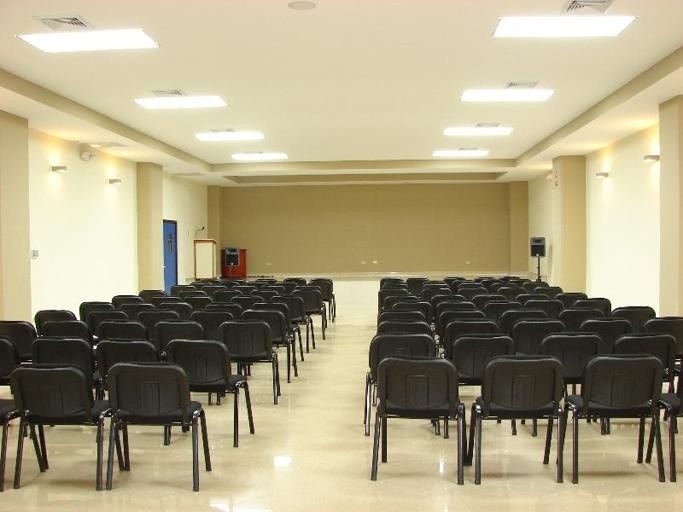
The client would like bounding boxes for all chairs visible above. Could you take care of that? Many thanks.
[162,340,255,448]
[370,359,468,485]
[647,358,683,482]
[1,338,46,493]
[564,354,679,483]
[10,366,110,491]
[467,358,565,486]
[106,362,212,491]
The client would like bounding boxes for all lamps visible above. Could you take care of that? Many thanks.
[642,155,660,161]
[108,178,122,184]
[595,172,609,178]
[52,165,66,172]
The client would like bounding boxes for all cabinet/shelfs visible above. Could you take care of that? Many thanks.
[194,239,217,281]
[221,248,247,278]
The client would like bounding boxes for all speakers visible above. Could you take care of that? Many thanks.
[530,237,546,257]
[225,247,241,267]
